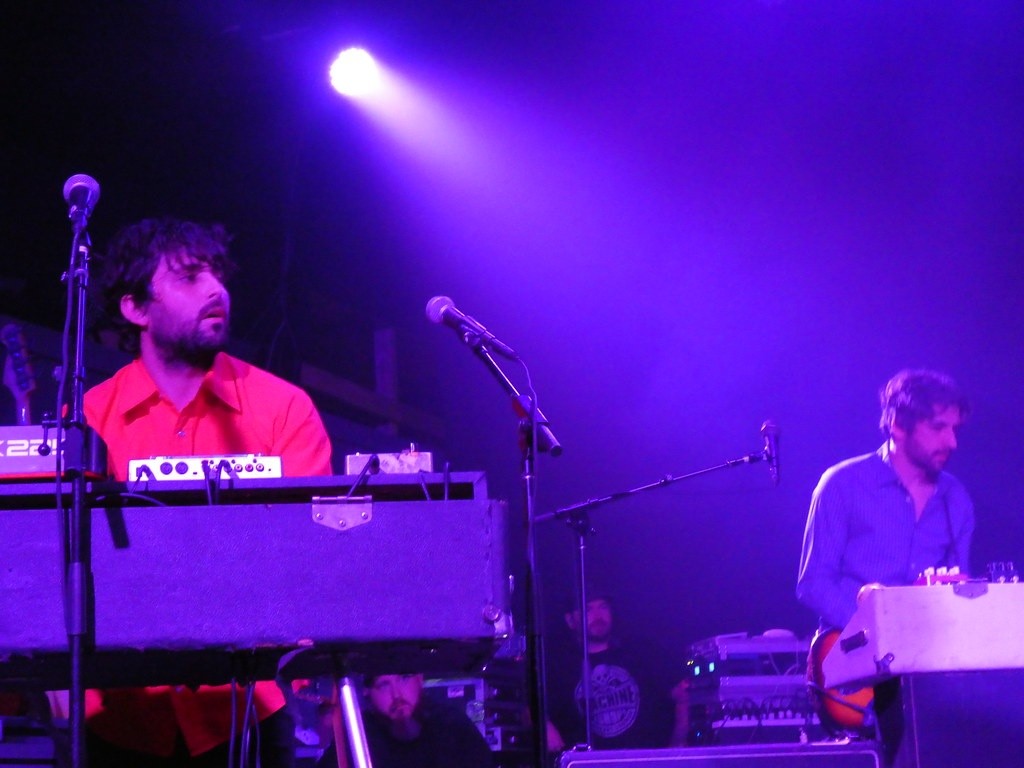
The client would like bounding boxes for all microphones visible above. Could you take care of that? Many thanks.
[425,294,519,362]
[761,420,782,487]
[61,173,101,232]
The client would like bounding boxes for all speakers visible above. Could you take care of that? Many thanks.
[559,737,882,768]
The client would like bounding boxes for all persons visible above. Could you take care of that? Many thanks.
[540,590,693,752]
[306,674,498,768]
[43,214,337,767]
[796,369,976,630]
